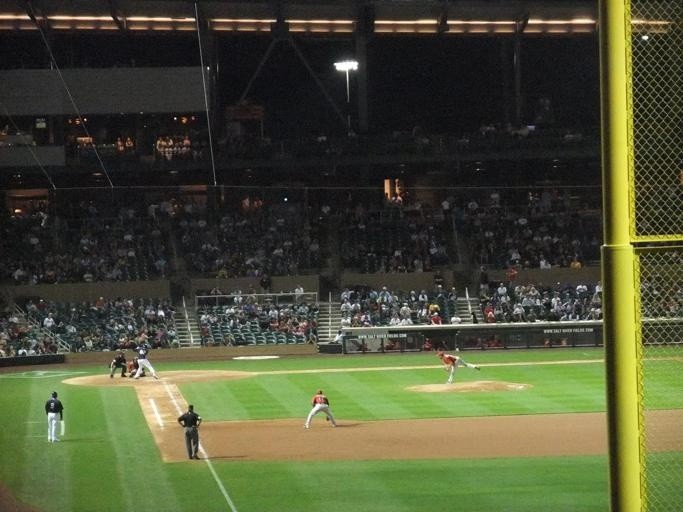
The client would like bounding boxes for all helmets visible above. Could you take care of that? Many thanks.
[318,390,322,394]
[52,392,57,397]
[188,405,192,410]
[438,352,443,356]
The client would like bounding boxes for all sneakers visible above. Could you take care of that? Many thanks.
[121,373,159,379]
[49,439,60,442]
[111,375,113,377]
[190,454,200,459]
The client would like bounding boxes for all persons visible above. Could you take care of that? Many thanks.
[177,405,202,460]
[304,389,336,429]
[45,392,64,442]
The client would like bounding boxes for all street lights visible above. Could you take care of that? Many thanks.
[334,56,360,136]
